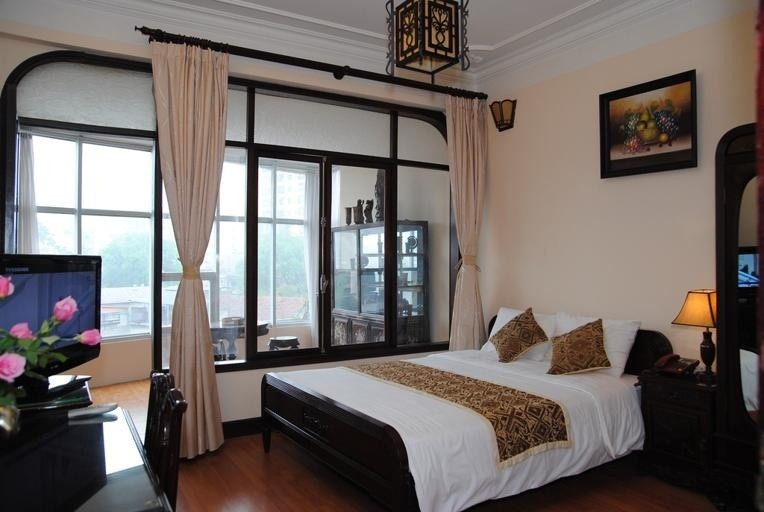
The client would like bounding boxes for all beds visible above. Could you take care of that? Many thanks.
[260,314,672,512]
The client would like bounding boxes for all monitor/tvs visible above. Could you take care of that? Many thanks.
[0,252,102,396]
[738,246,762,296]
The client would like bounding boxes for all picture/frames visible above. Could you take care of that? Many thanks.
[599,69,696,177]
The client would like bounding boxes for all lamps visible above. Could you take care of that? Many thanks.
[382,1,469,87]
[488,99,517,132]
[671,290,717,384]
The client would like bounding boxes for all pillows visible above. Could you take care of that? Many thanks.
[546,318,611,374]
[546,313,643,378]
[481,307,555,361]
[489,308,548,363]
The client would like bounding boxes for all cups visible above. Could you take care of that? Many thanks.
[377,242,383,253]
[345,207,351,225]
[353,206,361,224]
[350,258,355,269]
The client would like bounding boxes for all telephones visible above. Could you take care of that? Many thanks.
[652,354,700,376]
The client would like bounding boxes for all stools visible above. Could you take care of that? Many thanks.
[268,336,299,350]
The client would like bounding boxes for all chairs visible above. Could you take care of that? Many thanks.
[143,370,187,512]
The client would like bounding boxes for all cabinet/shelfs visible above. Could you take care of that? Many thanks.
[716,122,764,511]
[331,221,428,347]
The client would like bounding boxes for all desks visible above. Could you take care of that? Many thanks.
[207,316,268,361]
[1,406,173,512]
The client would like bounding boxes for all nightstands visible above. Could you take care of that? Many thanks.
[640,362,718,496]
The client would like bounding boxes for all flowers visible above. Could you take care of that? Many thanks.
[0,274,102,432]
